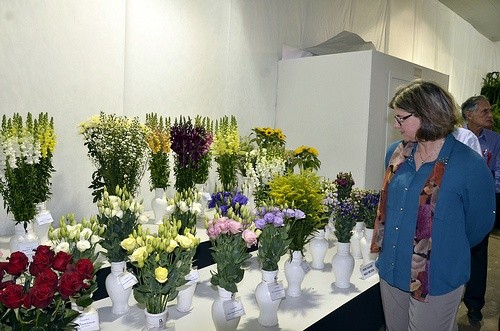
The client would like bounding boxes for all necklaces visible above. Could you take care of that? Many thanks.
[418,139,445,164]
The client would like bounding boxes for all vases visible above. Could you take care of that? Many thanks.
[151,188,167,224]
[139,307,169,331]
[360,228,378,262]
[212,284,241,331]
[284,249,307,297]
[332,241,355,288]
[351,222,366,259]
[32,202,51,245]
[309,229,329,270]
[10,220,39,263]
[176,264,197,313]
[196,184,208,220]
[105,261,132,314]
[255,270,285,327]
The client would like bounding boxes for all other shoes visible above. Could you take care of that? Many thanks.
[467,309,482,321]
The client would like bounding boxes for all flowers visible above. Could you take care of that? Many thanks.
[0,111,380,331]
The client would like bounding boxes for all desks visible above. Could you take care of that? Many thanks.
[0,212,387,331]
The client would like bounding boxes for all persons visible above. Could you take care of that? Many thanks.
[371,80,500,331]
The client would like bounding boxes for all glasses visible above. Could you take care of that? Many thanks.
[393,112,414,127]
[481,108,494,115]
